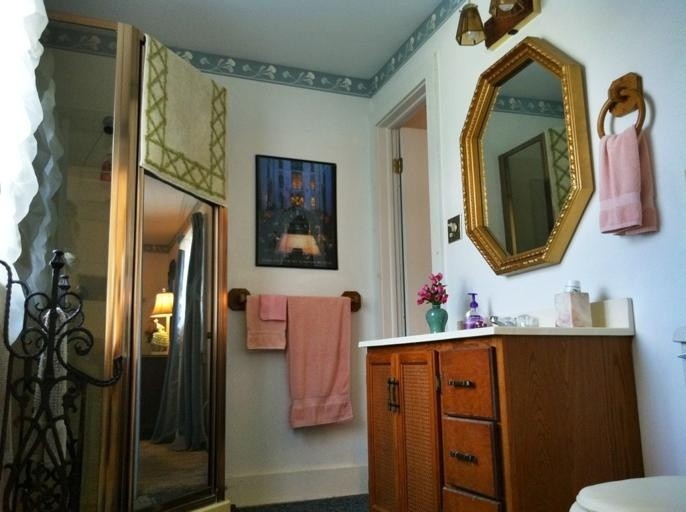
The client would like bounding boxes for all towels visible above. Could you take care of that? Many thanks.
[27,307,68,465]
[246,294,354,430]
[140,34,228,207]
[598,125,658,236]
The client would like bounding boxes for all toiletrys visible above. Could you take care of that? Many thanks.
[466,292,482,328]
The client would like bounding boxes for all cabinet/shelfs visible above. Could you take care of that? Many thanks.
[367,349,442,512]
[436,338,643,510]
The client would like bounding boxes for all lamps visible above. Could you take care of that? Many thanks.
[150,287,174,332]
[455,0,541,51]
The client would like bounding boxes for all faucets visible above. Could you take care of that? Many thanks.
[489,315,507,326]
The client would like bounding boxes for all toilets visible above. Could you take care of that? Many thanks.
[568,475,686,512]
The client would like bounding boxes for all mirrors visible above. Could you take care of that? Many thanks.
[122,170,228,512]
[459,36,594,276]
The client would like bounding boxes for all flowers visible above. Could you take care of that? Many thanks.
[416,274,448,304]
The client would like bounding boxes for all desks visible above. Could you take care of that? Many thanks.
[140,351,168,439]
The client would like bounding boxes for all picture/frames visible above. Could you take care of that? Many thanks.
[255,154,338,270]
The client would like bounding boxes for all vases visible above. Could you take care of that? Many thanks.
[426,303,448,333]
